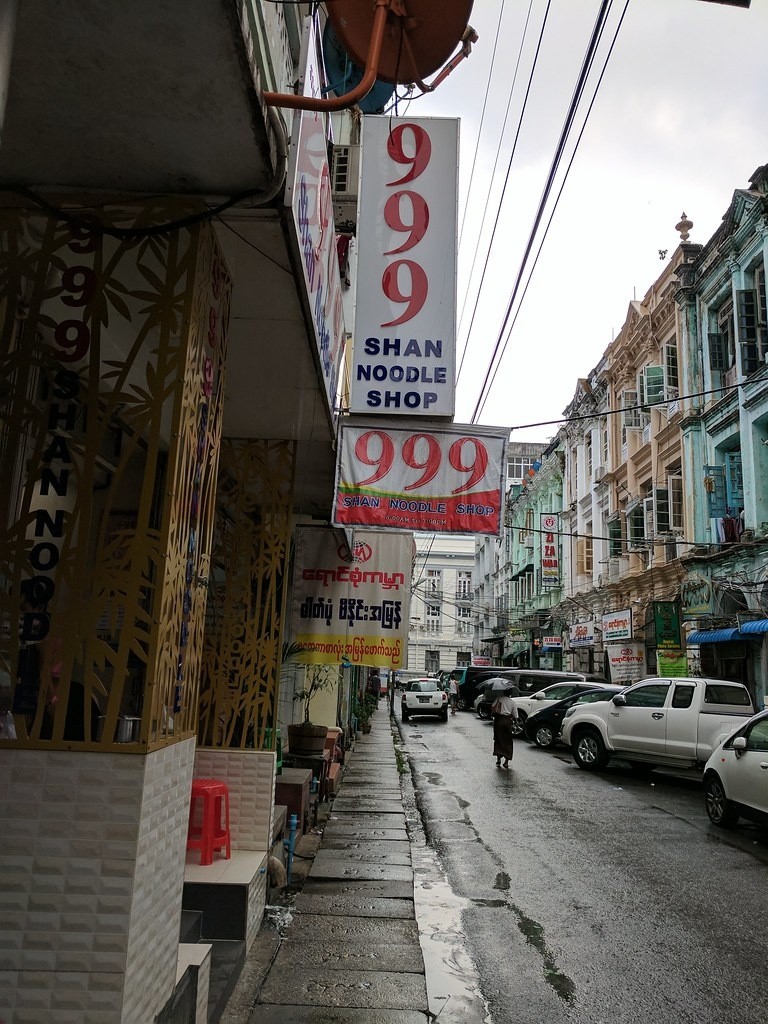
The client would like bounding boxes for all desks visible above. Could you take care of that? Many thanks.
[281,745,330,803]
[325,731,340,757]
[275,768,313,833]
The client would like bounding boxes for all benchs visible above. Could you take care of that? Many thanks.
[175,942,213,1024]
[273,804,287,843]
[184,746,277,957]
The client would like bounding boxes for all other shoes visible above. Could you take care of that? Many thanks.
[503,763,508,769]
[496,761,501,765]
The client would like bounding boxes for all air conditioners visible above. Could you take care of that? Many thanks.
[331,144,360,236]
[524,536,534,548]
[418,624,427,631]
[594,467,605,484]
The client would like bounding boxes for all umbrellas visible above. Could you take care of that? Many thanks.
[476,676,516,693]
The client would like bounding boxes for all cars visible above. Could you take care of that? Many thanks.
[702,707,768,827]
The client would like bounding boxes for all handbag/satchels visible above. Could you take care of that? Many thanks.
[491,696,501,718]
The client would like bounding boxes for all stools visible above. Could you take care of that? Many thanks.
[256,728,283,775]
[186,777,231,866]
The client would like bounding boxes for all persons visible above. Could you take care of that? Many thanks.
[448,673,460,715]
[492,687,519,770]
[368,670,381,710]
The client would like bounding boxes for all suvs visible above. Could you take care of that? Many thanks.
[434,667,666,772]
[402,678,448,722]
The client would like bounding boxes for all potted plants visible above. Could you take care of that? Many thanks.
[287,663,343,757]
[350,688,378,735]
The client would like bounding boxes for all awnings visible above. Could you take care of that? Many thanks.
[685,619,768,645]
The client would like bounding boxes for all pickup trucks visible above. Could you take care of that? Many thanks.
[559,675,768,785]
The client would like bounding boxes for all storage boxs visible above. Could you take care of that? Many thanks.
[318,763,341,793]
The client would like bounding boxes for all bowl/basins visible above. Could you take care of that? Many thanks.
[97,718,132,742]
[131,717,141,740]
